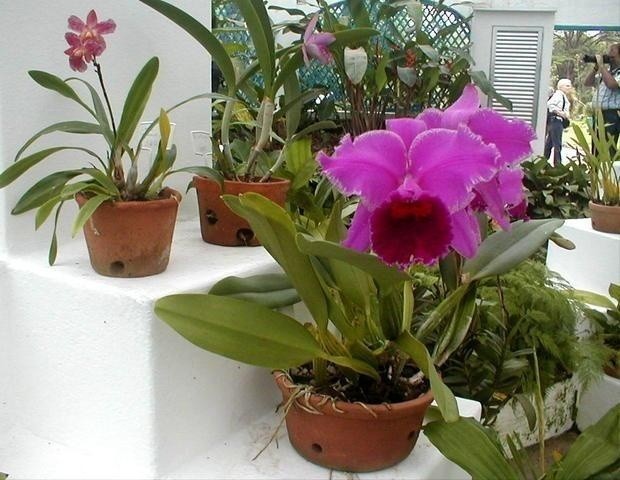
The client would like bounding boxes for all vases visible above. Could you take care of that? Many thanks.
[191,170,291,248]
[76,188,182,279]
[270,330,435,472]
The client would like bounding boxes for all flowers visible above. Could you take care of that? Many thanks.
[135,0,338,181]
[154,86,574,424]
[1,9,224,265]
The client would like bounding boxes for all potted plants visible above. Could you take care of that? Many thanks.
[573,109,620,232]
[564,282,620,436]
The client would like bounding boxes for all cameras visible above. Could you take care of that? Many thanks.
[583,54,610,64]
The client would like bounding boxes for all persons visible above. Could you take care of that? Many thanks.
[582,44,620,162]
[544,78,572,167]
